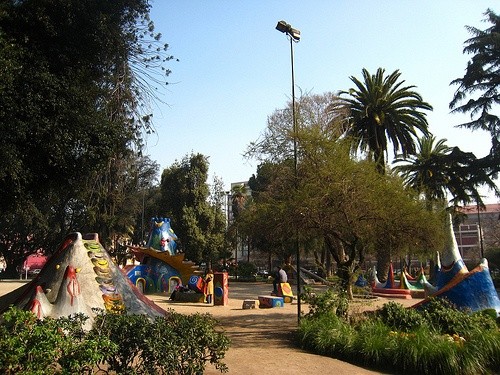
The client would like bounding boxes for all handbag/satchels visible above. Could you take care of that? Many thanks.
[206,275,212,282]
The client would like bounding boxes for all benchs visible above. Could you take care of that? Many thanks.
[257,295,283,308]
[243,299,256,308]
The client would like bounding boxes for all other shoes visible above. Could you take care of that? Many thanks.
[206,301,212,304]
[272,290,277,293]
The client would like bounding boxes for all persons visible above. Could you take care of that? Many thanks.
[272,266,288,294]
[201,270,214,303]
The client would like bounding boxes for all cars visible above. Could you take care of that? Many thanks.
[0,242,49,275]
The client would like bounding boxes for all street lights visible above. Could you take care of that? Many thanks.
[220,191,230,266]
[275,19,302,327]
[140,181,152,247]
